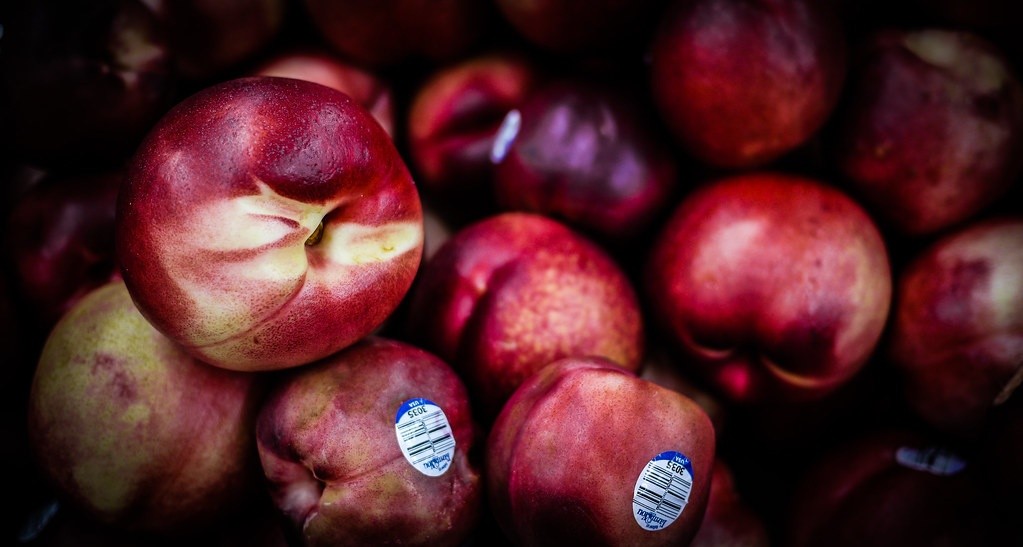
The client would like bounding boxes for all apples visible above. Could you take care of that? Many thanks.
[0,0,1023,547]
